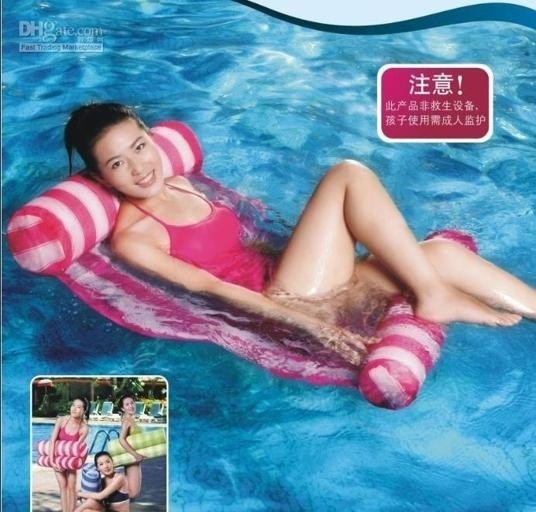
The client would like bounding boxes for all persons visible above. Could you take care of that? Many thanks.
[118,394,145,499]
[50,395,90,512]
[73,452,131,512]
[63,95,535,366]
